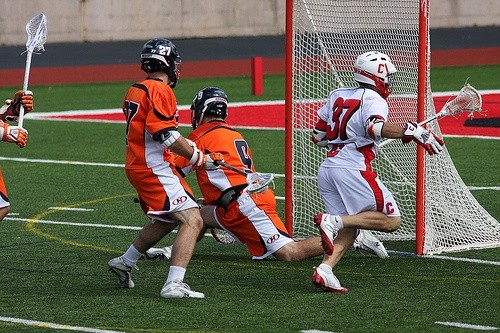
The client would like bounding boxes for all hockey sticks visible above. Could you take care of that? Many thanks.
[378,84,485,151]
[16,12,49,127]
[214,159,276,194]
[133,196,236,245]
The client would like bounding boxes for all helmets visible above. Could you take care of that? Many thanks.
[189,86,229,130]
[352,50,397,98]
[139,37,181,89]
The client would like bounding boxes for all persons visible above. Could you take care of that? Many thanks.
[310,50,445,294]
[0,90,33,222]
[108,37,224,299]
[144,87,390,262]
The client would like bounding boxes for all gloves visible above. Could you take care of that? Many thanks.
[2,124,28,148]
[402,119,447,158]
[11,89,33,117]
[189,145,224,172]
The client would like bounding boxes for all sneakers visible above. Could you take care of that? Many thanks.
[312,212,339,256]
[350,228,390,260]
[107,256,139,289]
[310,268,349,294]
[141,247,172,263]
[159,277,206,299]
